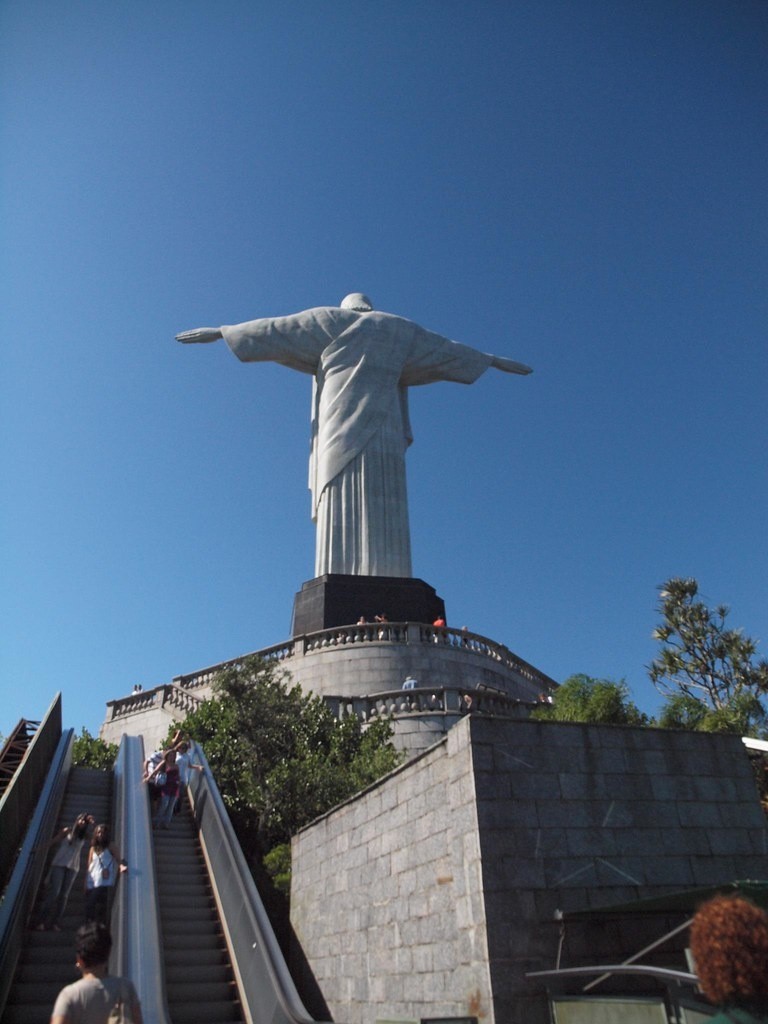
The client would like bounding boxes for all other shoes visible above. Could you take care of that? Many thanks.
[155,823,160,830]
[52,924,61,931]
[164,825,169,831]
[36,924,44,931]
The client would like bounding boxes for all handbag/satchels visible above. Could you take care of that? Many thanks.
[107,977,134,1024]
[155,761,168,786]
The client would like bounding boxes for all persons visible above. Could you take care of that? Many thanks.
[357,613,470,647]
[175,292,535,578]
[31,812,98,931]
[403,676,554,713]
[85,824,129,930]
[691,892,768,1024]
[143,730,206,828]
[132,684,143,694]
[51,932,143,1024]
